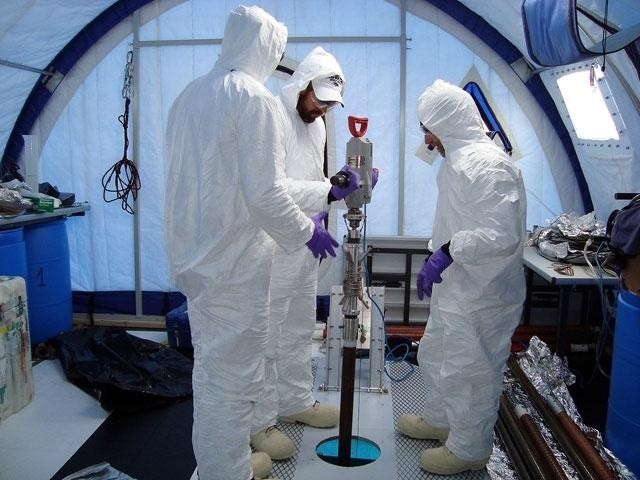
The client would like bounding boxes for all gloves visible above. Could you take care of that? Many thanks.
[372,168,379,189]
[331,165,360,200]
[305,212,339,258]
[417,248,452,300]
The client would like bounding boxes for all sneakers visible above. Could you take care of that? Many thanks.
[280,400,340,428]
[396,415,451,443]
[250,426,295,460]
[250,452,272,480]
[420,446,490,474]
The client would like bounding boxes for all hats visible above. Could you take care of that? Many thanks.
[311,75,344,108]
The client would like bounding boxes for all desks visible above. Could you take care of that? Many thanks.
[521,243,620,360]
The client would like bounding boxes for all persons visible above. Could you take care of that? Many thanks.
[250,46,378,462]
[395,77,528,476]
[161,3,340,480]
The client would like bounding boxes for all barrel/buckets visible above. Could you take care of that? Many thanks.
[27,220,74,346]
[0,227,27,280]
[606,292,640,473]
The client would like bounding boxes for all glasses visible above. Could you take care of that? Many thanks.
[310,90,339,111]
